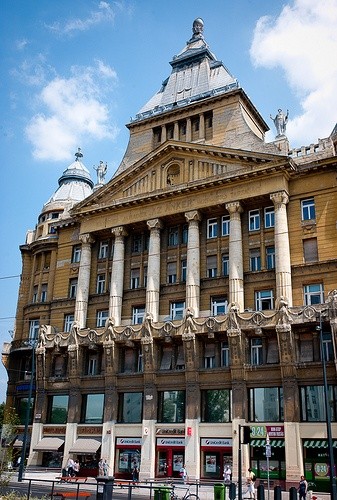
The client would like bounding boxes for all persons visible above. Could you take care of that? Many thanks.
[98,459,105,477]
[104,460,110,477]
[72,460,80,478]
[179,465,189,485]
[245,468,257,500]
[269,108,290,139]
[297,476,308,500]
[93,159,108,186]
[223,465,231,484]
[131,461,139,481]
[67,456,76,479]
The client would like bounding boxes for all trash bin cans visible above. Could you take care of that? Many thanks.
[257,485,264,500]
[289,487,297,500]
[154,489,171,500]
[229,483,236,500]
[214,483,225,500]
[63,468,68,482]
[274,486,281,500]
[95,476,115,500]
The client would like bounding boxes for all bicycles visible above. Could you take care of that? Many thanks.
[169,483,200,500]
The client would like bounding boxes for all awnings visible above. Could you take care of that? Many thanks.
[69,436,102,455]
[33,436,66,452]
[2,435,29,447]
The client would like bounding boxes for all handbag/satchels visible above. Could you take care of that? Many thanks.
[251,475,256,481]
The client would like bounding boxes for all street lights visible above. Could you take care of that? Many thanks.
[17,338,36,482]
[316,308,337,500]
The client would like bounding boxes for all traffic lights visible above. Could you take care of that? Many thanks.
[244,425,255,445]
